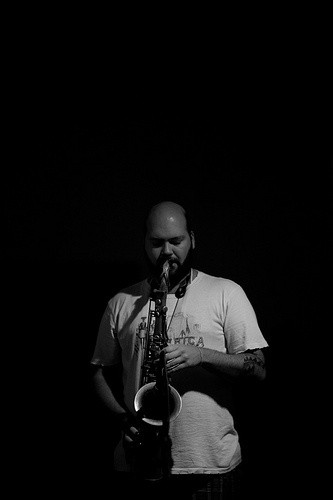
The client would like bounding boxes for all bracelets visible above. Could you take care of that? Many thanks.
[194,346,203,368]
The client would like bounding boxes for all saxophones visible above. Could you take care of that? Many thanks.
[135,261,182,482]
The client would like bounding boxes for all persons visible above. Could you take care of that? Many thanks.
[91,201,270,500]
